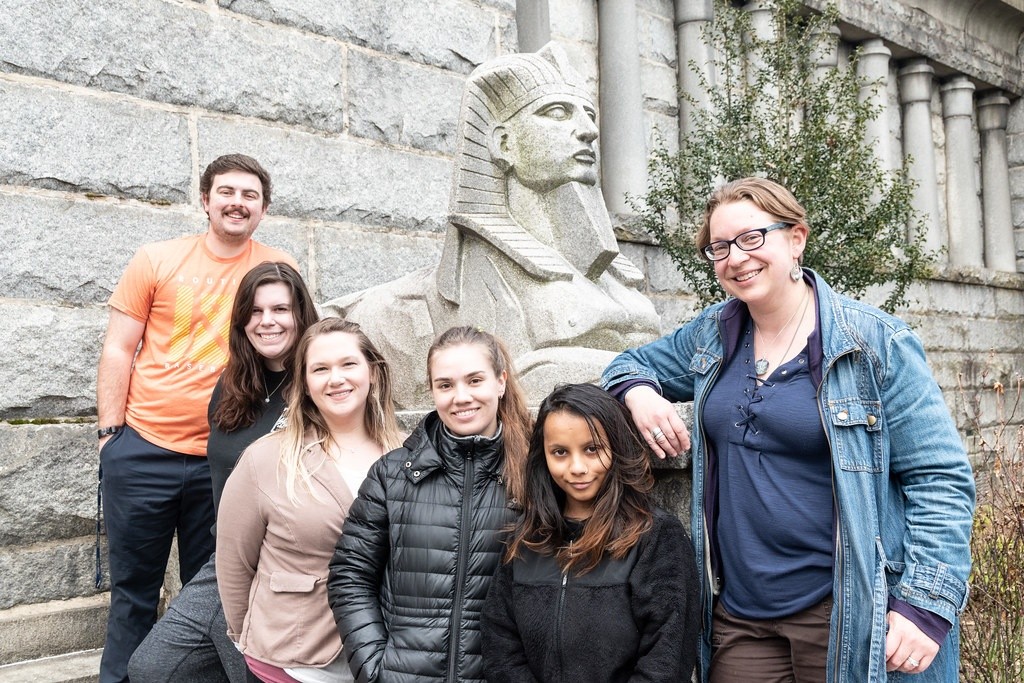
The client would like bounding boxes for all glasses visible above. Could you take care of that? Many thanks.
[701,222,788,262]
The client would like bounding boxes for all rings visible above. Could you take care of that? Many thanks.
[908,657,919,666]
[651,428,663,438]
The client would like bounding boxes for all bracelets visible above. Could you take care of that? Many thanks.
[97,426,121,439]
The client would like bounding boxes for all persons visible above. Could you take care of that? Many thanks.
[215,318,410,683]
[599,178,977,683]
[449,41,646,289]
[479,382,702,683]
[127,261,320,683]
[326,326,536,683]
[96,154,297,683]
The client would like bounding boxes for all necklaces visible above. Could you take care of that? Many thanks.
[263,371,289,402]
[755,284,809,376]
